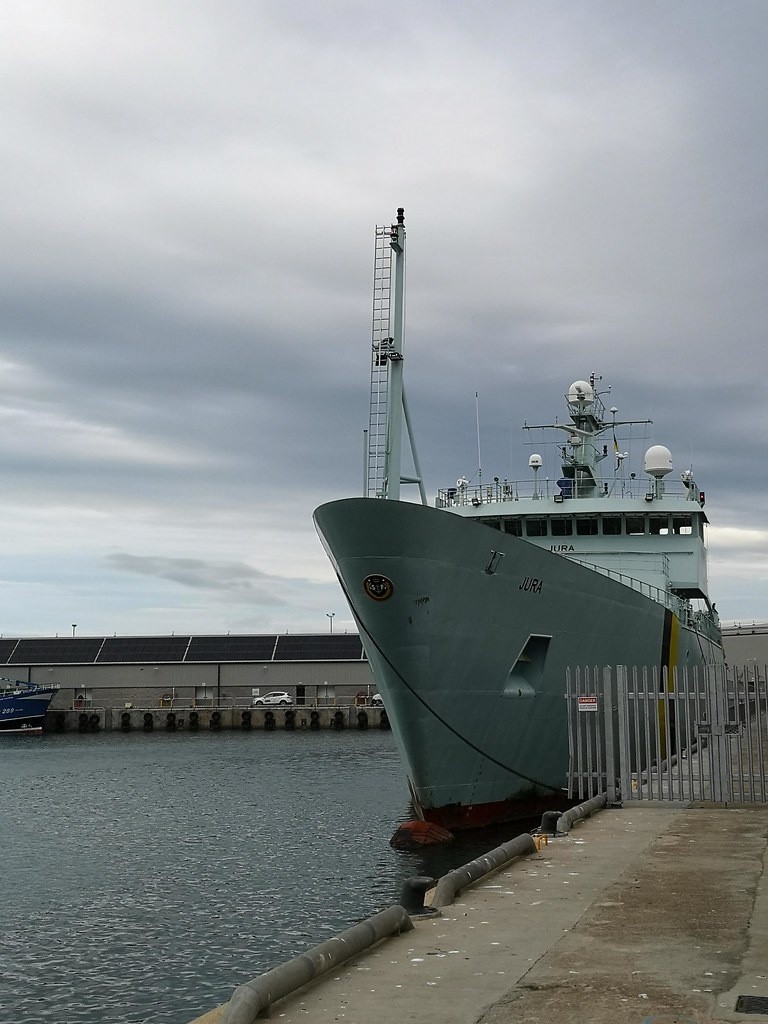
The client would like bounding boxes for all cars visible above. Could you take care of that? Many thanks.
[372,692,383,706]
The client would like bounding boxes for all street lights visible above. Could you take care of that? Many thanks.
[326,612,335,634]
[71,624,78,638]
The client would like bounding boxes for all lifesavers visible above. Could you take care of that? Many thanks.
[311,711,320,731]
[286,710,295,730]
[241,710,252,731]
[212,711,223,732]
[167,713,176,731]
[380,710,390,730]
[335,711,345,731]
[144,713,153,732]
[188,712,199,731]
[121,712,131,733]
[358,711,368,730]
[78,712,100,733]
[265,711,273,730]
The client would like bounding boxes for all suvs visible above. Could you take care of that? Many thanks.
[252,691,293,706]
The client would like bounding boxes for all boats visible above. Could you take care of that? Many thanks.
[311,206,727,851]
[0,676,61,732]
